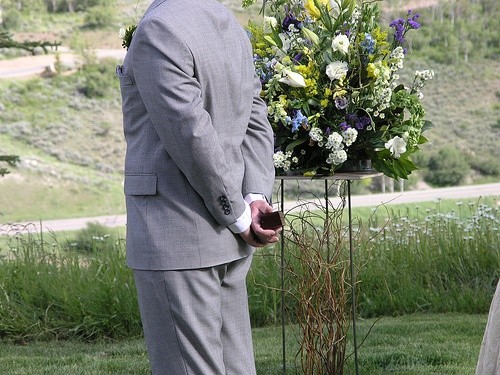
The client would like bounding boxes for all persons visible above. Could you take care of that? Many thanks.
[115,1,278,374]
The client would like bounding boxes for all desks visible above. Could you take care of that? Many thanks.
[273,171,385,375]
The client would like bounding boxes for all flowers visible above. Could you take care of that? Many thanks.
[118,24,138,52]
[239,0,436,185]
